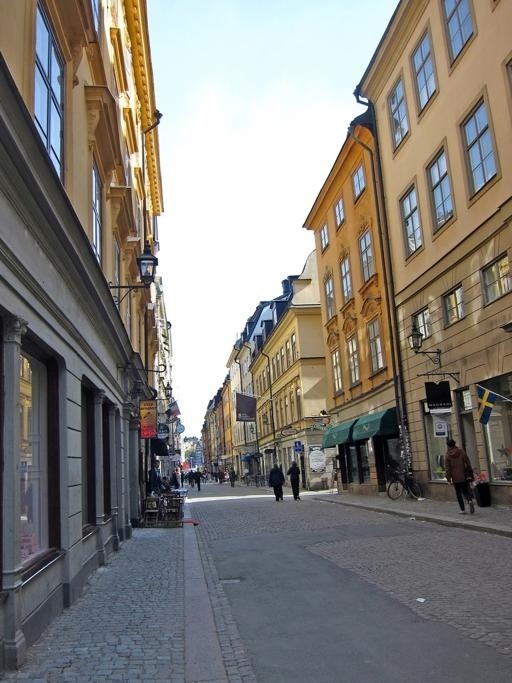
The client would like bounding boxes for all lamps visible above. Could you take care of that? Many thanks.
[108,240,158,305]
[406,322,442,368]
[262,413,272,425]
[154,381,173,401]
[130,378,143,394]
[249,423,256,434]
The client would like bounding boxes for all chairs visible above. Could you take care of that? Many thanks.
[141,490,184,528]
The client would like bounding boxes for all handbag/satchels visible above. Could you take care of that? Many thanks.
[464,466,474,482]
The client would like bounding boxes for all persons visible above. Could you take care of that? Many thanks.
[161,467,236,493]
[444,439,475,514]
[287,461,301,501]
[269,464,285,501]
[148,460,161,497]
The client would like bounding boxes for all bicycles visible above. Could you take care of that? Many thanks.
[383,463,423,501]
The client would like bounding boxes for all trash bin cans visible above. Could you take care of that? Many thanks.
[474,482,491,508]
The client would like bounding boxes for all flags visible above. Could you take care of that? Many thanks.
[475,387,497,425]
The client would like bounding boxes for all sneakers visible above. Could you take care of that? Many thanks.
[458,510,465,514]
[469,501,475,514]
